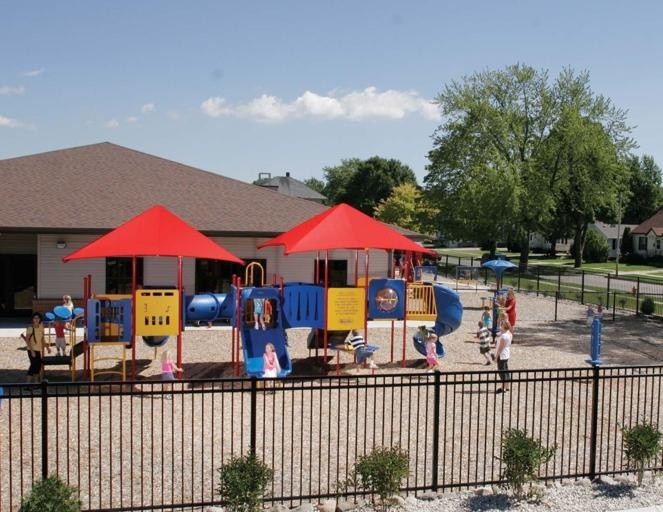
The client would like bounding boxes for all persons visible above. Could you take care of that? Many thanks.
[159,349,184,400]
[348,329,379,369]
[497,311,508,329]
[480,305,491,329]
[493,295,505,334]
[472,320,494,365]
[264,300,272,323]
[54,317,75,357]
[252,298,267,332]
[62,294,74,329]
[494,321,513,394]
[423,333,440,371]
[25,311,51,391]
[416,325,428,343]
[262,341,281,395]
[498,286,516,333]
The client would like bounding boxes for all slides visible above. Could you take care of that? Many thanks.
[412,284,463,358]
[241,324,292,380]
